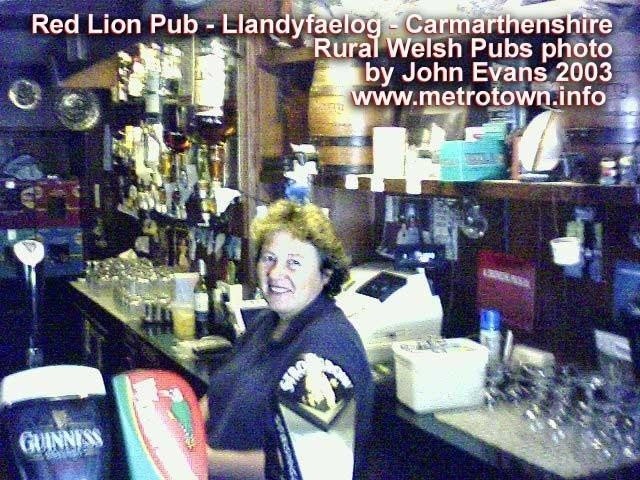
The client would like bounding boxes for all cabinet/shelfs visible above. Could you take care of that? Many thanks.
[255,0,640,380]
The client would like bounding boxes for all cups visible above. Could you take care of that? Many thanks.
[85,256,173,314]
[485,364,640,472]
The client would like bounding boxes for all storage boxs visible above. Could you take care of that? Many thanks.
[391,335,489,414]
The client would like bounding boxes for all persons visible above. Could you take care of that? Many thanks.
[196,198,377,480]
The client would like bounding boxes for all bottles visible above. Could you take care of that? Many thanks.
[194,259,214,341]
[110,37,236,224]
[479,305,503,361]
[141,304,172,336]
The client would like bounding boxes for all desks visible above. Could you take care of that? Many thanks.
[64,276,239,404]
[385,393,638,480]
